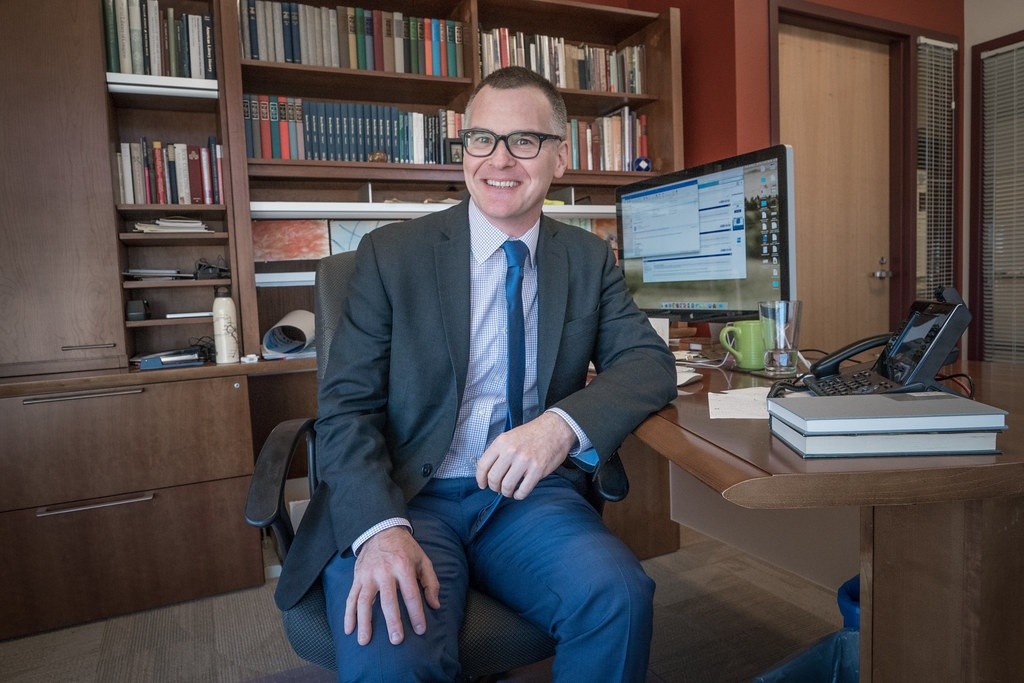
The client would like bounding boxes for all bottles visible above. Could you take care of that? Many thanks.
[211,288,239,364]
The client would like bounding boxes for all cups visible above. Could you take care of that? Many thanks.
[720,320,774,369]
[758,299,803,374]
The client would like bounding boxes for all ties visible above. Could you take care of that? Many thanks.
[462,242,529,545]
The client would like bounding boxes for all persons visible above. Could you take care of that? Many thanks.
[274,65,679,683]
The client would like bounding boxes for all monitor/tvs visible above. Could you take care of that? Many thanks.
[615,145,799,362]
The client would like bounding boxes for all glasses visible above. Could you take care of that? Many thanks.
[457,128,563,159]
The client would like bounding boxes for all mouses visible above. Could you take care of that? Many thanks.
[676,372,703,387]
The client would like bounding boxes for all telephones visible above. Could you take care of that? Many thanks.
[802,284,972,398]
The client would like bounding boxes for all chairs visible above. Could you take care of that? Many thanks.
[242,251,630,683]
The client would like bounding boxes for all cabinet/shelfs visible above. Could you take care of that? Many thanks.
[0,0,686,645]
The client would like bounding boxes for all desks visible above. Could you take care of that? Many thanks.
[586,336,1024,683]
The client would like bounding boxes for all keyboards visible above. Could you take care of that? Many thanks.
[677,366,695,373]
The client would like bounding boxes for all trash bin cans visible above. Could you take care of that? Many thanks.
[837,573,860,628]
[750,629,859,682]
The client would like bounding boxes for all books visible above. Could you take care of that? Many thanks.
[767,390,1010,461]
[122,268,195,283]
[565,105,648,172]
[167,312,212,317]
[237,0,465,78]
[478,27,644,94]
[243,93,466,165]
[133,218,216,234]
[105,0,215,79]
[115,134,225,207]
